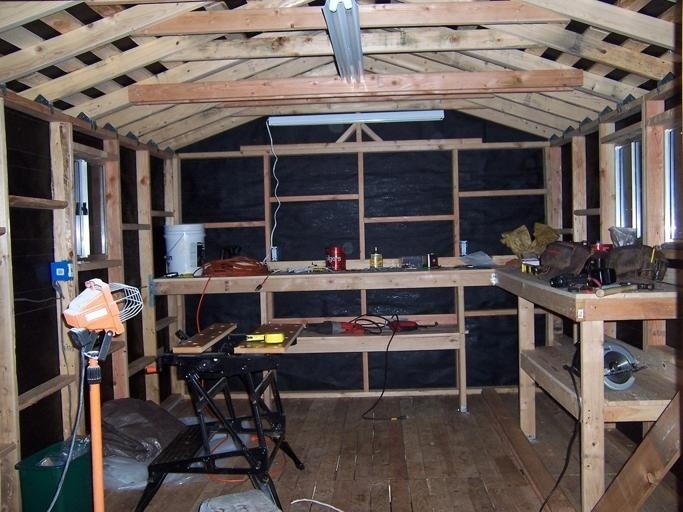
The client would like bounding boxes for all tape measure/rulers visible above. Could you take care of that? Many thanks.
[244,333,285,344]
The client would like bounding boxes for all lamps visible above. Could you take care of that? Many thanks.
[321,0,364,84]
[268,110,445,128]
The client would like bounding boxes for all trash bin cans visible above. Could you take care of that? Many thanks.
[15,437,93,512]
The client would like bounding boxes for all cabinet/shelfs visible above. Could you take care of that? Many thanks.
[137,267,682,512]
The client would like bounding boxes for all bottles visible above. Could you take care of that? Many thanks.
[371,245,382,269]
[324,246,345,273]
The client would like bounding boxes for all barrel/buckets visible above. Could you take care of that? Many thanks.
[165,223,207,276]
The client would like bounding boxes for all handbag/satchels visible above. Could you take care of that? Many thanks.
[541,241,668,283]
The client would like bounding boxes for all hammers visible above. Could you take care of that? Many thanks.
[595,282,653,297]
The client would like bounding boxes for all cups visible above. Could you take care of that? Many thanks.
[400,255,423,268]
